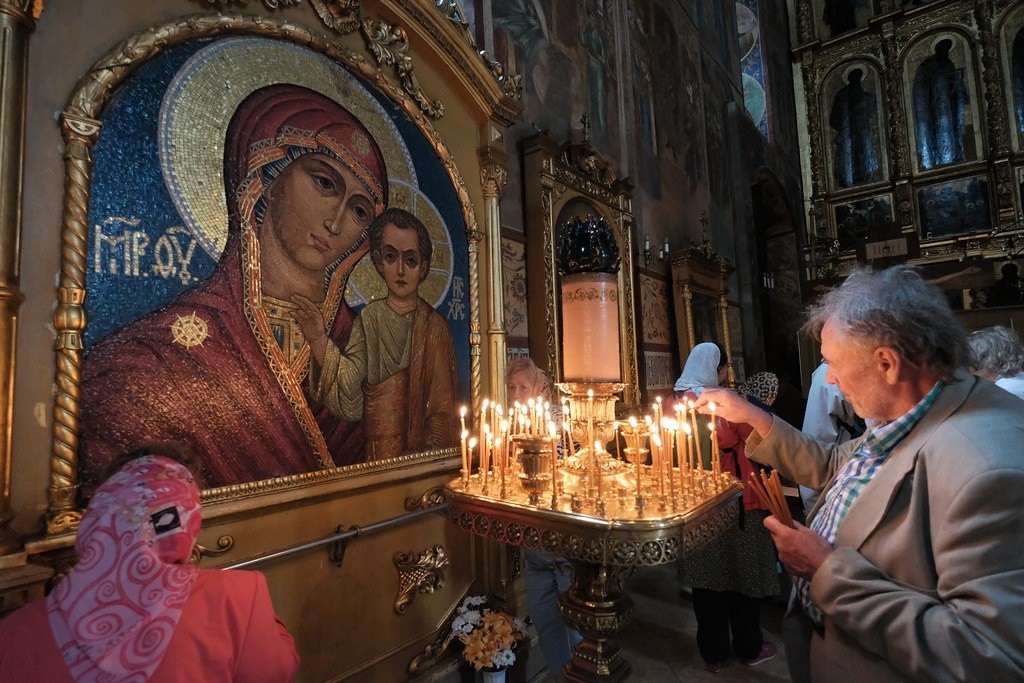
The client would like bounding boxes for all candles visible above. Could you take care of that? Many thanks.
[481,388,721,495]
[468,437,476,476]
[461,432,468,469]
[461,406,467,439]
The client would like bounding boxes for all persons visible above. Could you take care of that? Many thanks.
[686,264,1024,683]
[0,453,301,683]
[798,357,867,515]
[659,342,777,673]
[965,323,1024,402]
[731,371,779,415]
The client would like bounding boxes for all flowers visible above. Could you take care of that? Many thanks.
[451,596,527,671]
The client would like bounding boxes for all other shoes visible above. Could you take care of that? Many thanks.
[703,662,721,672]
[745,641,777,666]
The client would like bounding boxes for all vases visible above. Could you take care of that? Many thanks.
[481,665,509,683]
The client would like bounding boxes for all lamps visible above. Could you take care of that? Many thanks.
[562,272,622,384]
